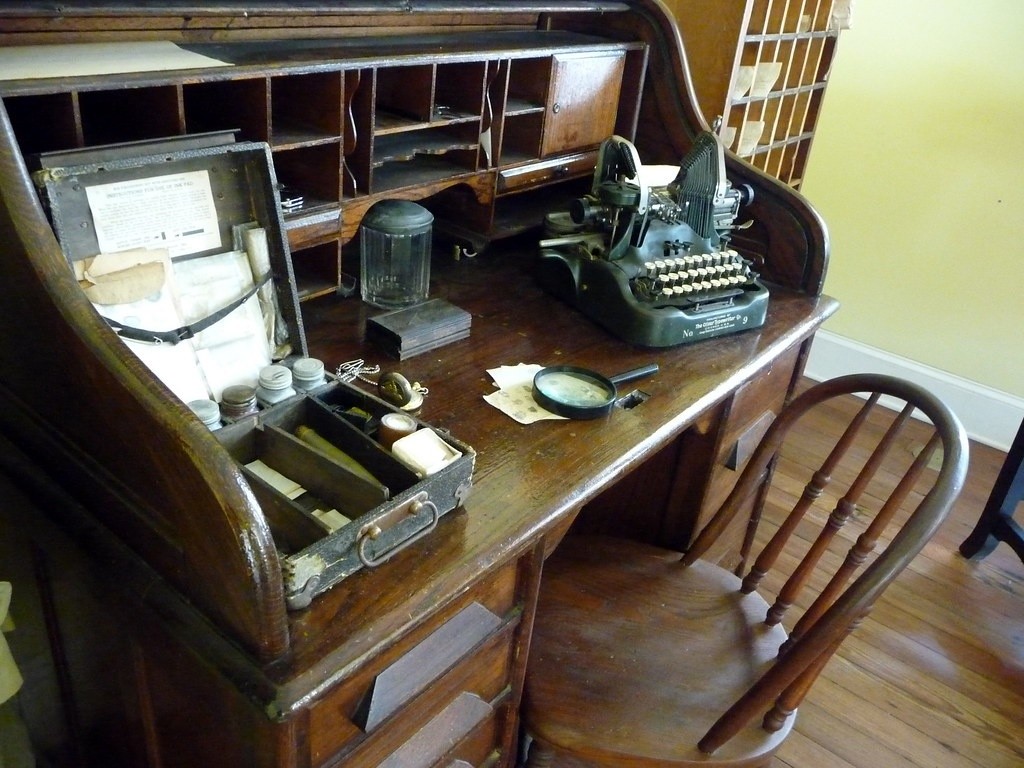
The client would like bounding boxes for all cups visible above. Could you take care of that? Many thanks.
[361,199,434,311]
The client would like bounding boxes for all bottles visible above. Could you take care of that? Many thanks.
[254,365,297,408]
[187,399,223,434]
[220,384,259,425]
[293,357,327,390]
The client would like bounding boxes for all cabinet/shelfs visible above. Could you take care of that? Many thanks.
[665,0,840,192]
[0,0,837,768]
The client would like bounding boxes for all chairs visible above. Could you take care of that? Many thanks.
[520,373,969,768]
